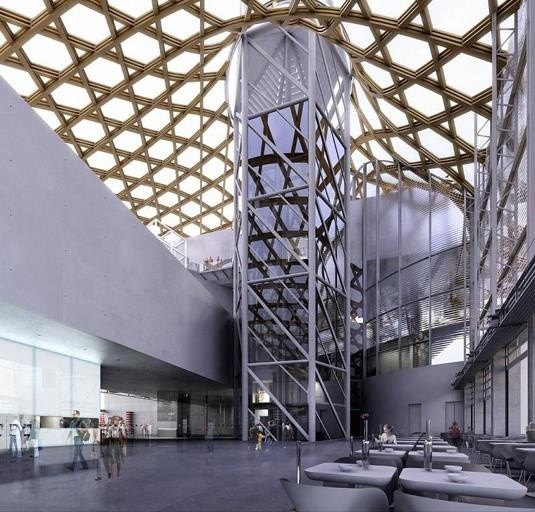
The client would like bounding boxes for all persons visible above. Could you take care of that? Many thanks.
[0,405,296,484]
[448,421,463,447]
[380,423,398,444]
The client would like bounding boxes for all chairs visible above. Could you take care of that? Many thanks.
[278,432,535,512]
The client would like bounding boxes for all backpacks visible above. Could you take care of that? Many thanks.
[77,427,91,442]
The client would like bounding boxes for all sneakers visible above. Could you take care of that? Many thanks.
[80,466,90,471]
[94,476,102,481]
[116,473,121,480]
[107,476,113,484]
[66,465,75,472]
